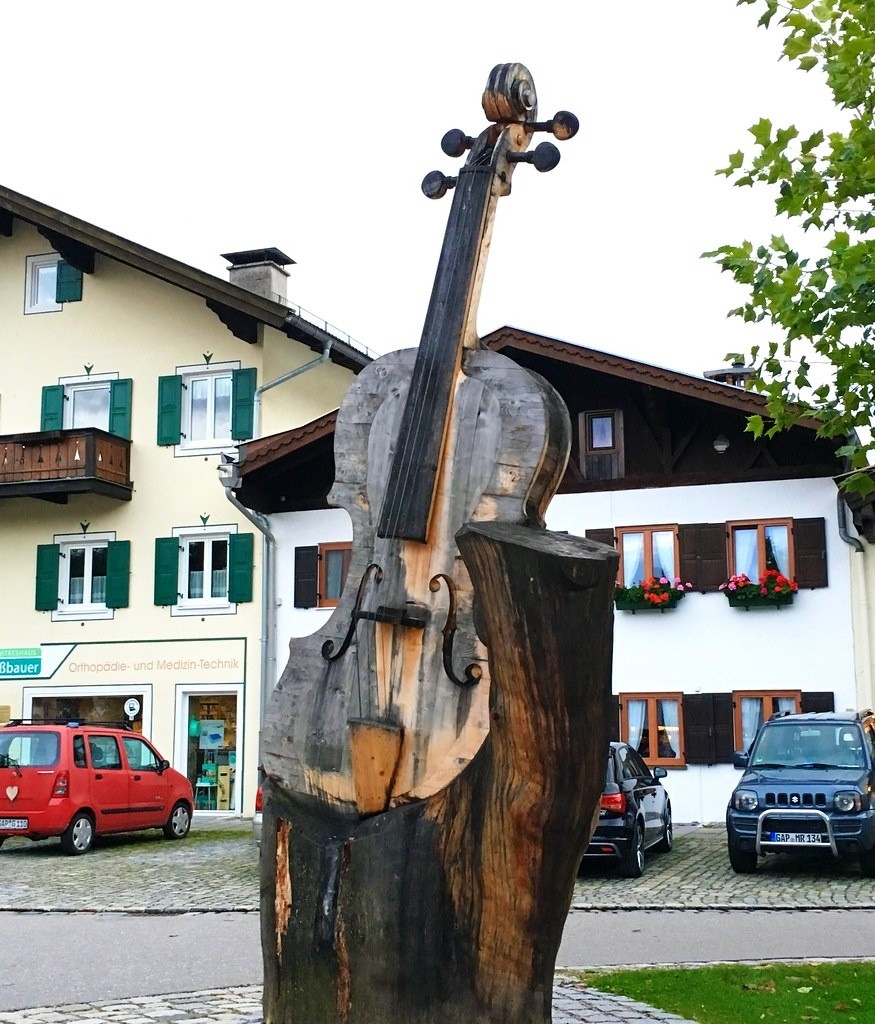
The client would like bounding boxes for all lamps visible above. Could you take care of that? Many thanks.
[712,415,730,455]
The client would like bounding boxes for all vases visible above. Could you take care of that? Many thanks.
[615,599,677,614]
[728,596,793,611]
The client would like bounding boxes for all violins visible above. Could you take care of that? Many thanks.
[251,62,579,815]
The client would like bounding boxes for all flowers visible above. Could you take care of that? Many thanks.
[719,570,798,599]
[614,577,693,607]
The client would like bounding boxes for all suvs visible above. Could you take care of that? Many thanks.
[725,709,875,878]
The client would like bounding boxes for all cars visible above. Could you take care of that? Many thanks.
[0,718,195,856]
[584,741,673,878]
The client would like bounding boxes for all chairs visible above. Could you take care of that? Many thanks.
[838,728,863,765]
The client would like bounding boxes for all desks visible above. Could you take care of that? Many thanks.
[194,783,218,810]
